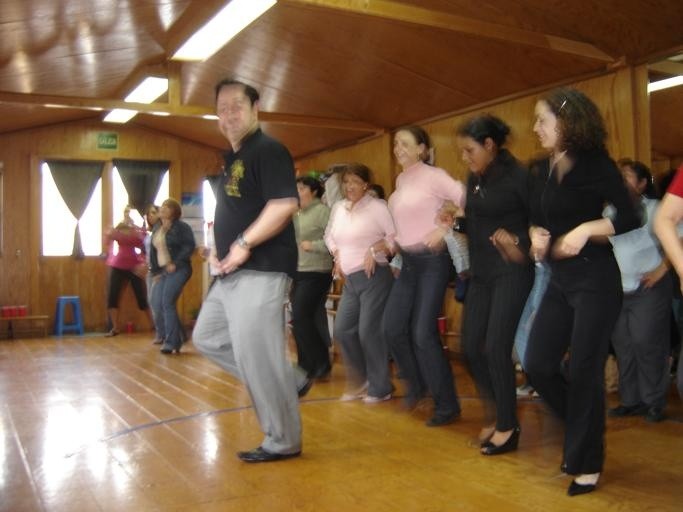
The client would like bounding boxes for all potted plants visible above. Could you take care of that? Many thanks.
[188,307,200,329]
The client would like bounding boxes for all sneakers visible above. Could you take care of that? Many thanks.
[341,383,370,399]
[362,388,392,404]
[517,387,537,401]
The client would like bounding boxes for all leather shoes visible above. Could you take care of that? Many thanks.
[293,380,313,397]
[106,328,121,337]
[610,404,643,418]
[642,407,664,422]
[428,412,460,425]
[237,445,301,461]
[403,392,429,408]
[304,368,334,380]
[154,339,163,344]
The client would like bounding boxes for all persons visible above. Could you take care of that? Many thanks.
[323,163,395,402]
[143,205,163,319]
[150,199,196,353]
[514,257,551,398]
[286,177,330,397]
[106,206,154,337]
[602,159,677,422]
[366,184,386,199]
[651,160,683,292]
[388,127,466,427]
[524,89,647,497]
[457,115,540,454]
[191,80,314,463]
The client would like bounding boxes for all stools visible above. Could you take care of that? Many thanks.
[54,296,84,335]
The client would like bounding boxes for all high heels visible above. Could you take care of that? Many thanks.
[568,467,604,495]
[159,342,183,354]
[481,424,521,455]
[469,421,498,449]
[561,461,582,474]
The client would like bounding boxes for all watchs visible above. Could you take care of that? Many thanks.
[235,232,254,253]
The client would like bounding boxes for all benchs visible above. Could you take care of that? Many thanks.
[0,315,49,340]
